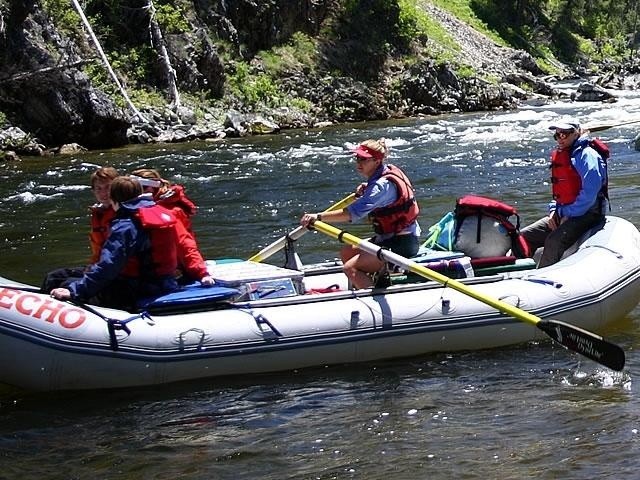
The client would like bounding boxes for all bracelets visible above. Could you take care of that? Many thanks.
[316,213,322,222]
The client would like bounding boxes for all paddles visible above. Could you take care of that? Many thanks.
[147,276,505,317]
[306,217,626,371]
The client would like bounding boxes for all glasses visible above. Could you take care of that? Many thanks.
[553,130,572,141]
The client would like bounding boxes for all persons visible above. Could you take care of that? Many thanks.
[40,168,120,294]
[299,140,422,291]
[132,168,216,287]
[49,175,177,314]
[509,122,610,268]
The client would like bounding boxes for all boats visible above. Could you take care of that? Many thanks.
[0,215,640,392]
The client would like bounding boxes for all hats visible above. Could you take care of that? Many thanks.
[548,118,580,129]
[349,145,385,159]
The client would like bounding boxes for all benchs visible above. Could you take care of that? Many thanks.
[394,246,465,283]
[144,281,240,315]
